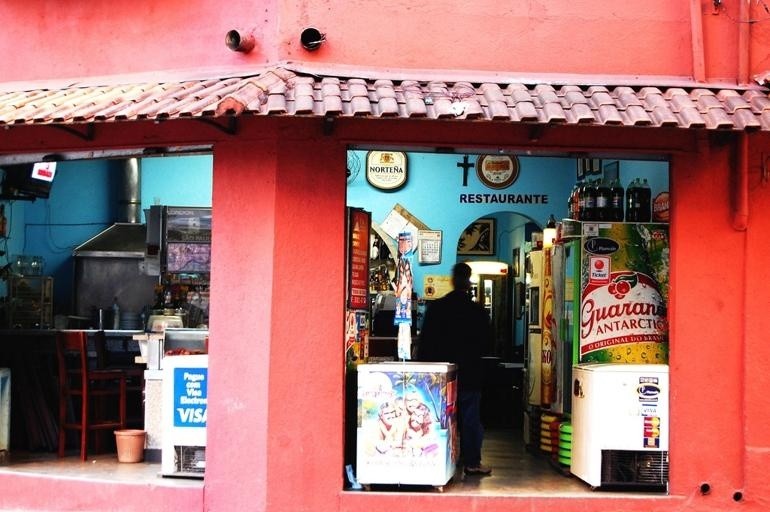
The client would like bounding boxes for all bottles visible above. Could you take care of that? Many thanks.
[581,223,670,366]
[110,297,120,330]
[567,176,654,223]
[546,215,557,229]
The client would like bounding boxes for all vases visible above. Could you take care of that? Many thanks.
[113,430,147,463]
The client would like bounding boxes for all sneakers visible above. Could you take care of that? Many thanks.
[464,464,492,475]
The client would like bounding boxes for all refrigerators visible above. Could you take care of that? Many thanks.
[355,360,461,487]
[522,220,669,495]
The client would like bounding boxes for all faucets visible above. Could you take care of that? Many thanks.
[98,307,105,330]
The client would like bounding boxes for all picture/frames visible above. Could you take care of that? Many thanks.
[577,157,619,181]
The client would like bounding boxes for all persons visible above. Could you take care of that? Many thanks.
[418,263,499,476]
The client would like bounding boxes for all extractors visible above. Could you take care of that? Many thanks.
[71,157,148,259]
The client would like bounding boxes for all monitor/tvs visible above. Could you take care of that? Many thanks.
[8,161,57,198]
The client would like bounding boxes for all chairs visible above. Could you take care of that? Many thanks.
[56,332,145,462]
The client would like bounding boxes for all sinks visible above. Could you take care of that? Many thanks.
[61,327,145,334]
